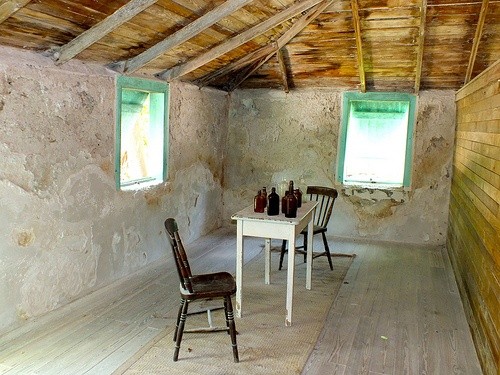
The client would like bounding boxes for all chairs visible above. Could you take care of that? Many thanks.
[279,186,338,271]
[164,218,240,364]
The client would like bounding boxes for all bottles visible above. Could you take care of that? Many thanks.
[282,180,302,218]
[254,186,267,213]
[267,187,280,216]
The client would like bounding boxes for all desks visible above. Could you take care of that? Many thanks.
[231,196,320,326]
